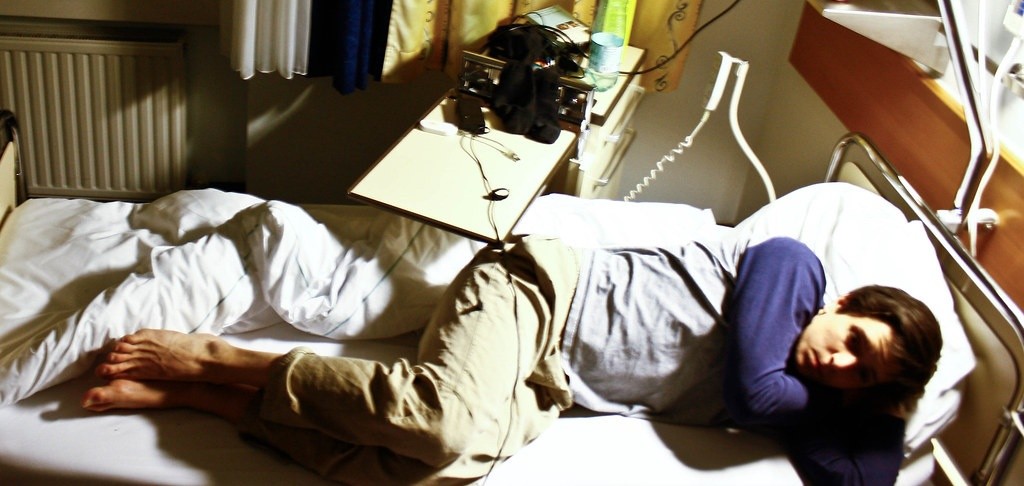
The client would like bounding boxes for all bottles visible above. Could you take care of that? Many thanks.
[585,0,628,92]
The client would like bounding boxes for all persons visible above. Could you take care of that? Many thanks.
[82,234,943,485]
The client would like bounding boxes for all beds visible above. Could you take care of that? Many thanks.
[0,122,1024,486]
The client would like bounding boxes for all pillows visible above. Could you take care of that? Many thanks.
[733,181,963,461]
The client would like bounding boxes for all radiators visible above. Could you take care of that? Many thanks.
[0,33,189,205]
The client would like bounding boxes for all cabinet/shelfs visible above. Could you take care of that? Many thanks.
[349,41,647,248]
